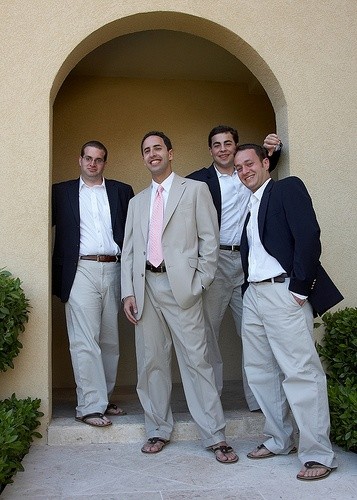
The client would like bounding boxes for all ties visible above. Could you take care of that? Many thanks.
[148,184,164,269]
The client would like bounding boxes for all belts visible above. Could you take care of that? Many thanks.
[220,244,240,251]
[145,264,166,273]
[81,254,121,263]
[253,272,289,283]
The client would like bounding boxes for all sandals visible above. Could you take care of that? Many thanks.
[297,460,338,481]
[141,437,171,453]
[247,443,298,459]
[209,443,239,464]
[104,404,127,416]
[75,412,112,427]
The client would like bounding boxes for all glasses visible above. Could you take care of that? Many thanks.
[83,156,105,165]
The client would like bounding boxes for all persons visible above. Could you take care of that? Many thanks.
[233,143,345,481]
[120,131,240,463]
[185,126,283,412]
[52,141,135,428]
